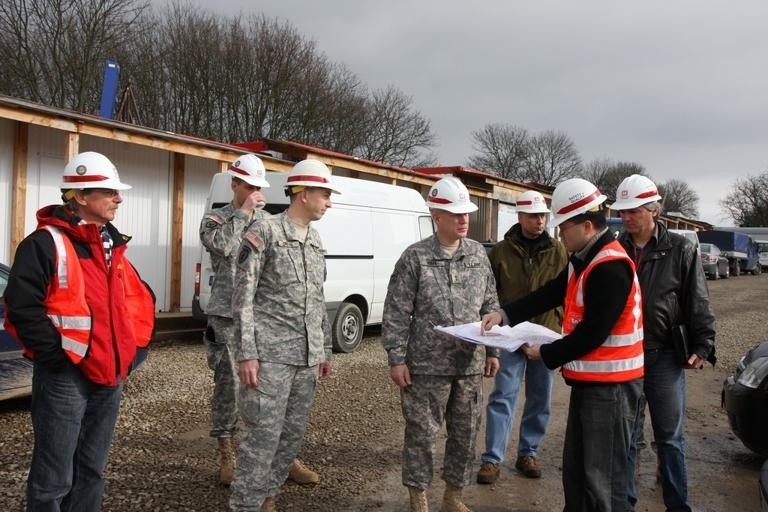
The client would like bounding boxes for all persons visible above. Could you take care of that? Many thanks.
[477,190,570,483]
[199,154,319,484]
[380,175,500,512]
[2,150,155,512]
[480,179,644,512]
[226,159,334,512]
[608,174,716,512]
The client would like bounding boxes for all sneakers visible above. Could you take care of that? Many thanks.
[476,462,501,483]
[514,454,542,477]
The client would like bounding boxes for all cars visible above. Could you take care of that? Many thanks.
[700,242,730,280]
[720,337,768,458]
[0,263,150,403]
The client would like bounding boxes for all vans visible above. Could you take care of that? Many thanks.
[755,240,768,268]
[668,228,701,256]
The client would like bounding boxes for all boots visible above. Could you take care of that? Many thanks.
[407,485,428,512]
[441,481,473,512]
[288,458,318,485]
[216,436,237,484]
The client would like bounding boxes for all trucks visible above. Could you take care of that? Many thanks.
[191,171,437,354]
[696,230,762,277]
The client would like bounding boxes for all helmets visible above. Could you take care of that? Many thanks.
[60,150,133,191]
[226,153,271,188]
[546,178,608,229]
[425,176,480,214]
[516,190,551,214]
[283,158,342,195]
[609,174,662,211]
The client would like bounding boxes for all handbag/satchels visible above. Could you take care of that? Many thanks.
[671,321,693,366]
